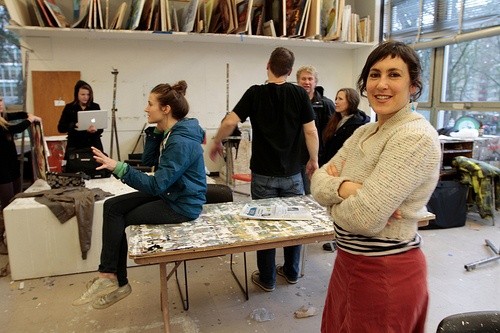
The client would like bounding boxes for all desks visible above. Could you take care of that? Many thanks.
[125,193,435,333]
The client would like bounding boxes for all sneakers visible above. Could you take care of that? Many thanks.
[275,265,298,284]
[72,276,119,305]
[91,284,132,309]
[250,270,275,293]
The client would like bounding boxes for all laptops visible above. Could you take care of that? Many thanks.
[74,110,108,131]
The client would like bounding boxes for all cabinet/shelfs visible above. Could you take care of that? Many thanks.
[2,177,139,280]
[439,139,475,181]
[4,1,378,49]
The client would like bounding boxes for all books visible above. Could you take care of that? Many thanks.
[32,0,371,42]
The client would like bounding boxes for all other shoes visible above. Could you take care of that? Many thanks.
[0,241,8,255]
[322,242,337,251]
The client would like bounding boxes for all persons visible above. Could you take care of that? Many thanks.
[57,80,104,172]
[0,115,39,254]
[81,81,207,309]
[209,47,319,291]
[296,64,370,250]
[310,42,441,333]
